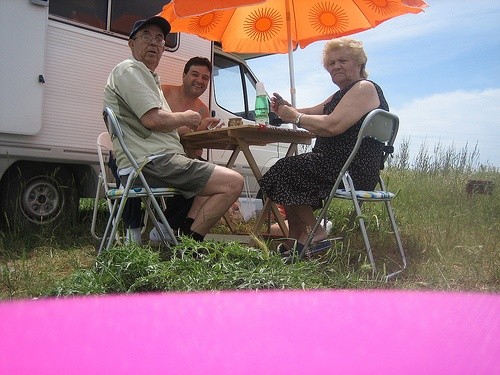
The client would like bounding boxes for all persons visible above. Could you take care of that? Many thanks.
[102,15,245,259]
[120,55,214,246]
[257,38,389,258]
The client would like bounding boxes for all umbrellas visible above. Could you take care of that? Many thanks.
[157,0,428,156]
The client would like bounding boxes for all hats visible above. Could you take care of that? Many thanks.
[129,16,172,39]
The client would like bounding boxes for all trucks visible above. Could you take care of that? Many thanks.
[1,0,315,235]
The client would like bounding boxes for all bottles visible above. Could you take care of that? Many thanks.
[255,95,269,125]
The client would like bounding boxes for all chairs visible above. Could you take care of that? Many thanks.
[296,109,412,282]
[90,130,175,241]
[97,106,190,261]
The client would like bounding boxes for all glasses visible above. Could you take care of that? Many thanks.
[132,32,166,47]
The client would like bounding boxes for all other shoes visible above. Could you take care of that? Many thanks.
[178,242,207,258]
[149,222,178,247]
[124,228,142,245]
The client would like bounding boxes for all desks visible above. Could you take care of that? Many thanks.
[180,126,314,254]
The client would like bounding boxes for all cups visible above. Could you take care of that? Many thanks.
[255,82,268,96]
[228,118,243,127]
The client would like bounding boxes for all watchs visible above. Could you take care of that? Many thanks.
[295,113,304,128]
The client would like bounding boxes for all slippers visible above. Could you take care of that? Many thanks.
[283,240,331,258]
